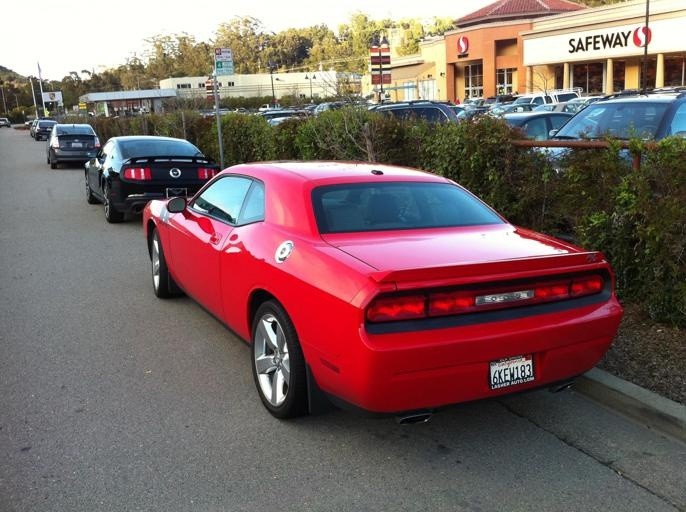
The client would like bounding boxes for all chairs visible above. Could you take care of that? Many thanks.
[367,194,399,229]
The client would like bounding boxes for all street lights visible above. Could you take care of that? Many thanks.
[369,28,390,93]
[266,62,275,108]
[305,74,316,99]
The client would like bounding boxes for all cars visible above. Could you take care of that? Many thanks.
[0,117,10,128]
